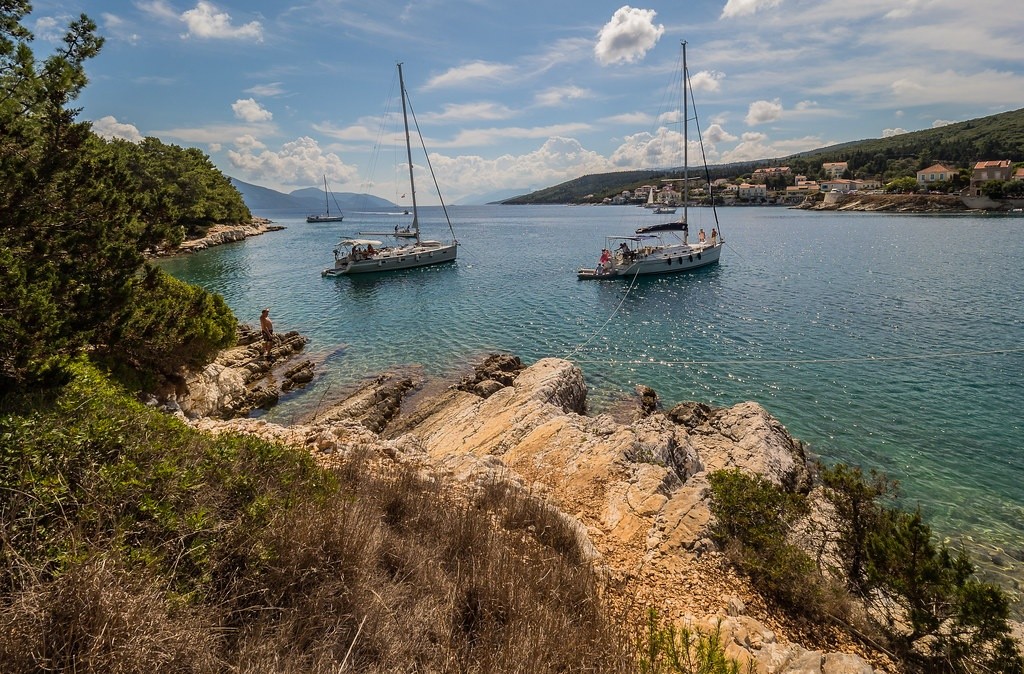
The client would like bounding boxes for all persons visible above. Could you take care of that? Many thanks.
[614,242,635,263]
[352,243,377,262]
[711,228,718,247]
[594,249,614,275]
[394,224,399,235]
[698,229,705,246]
[260,308,274,358]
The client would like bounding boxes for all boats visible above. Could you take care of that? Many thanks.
[577,268,612,277]
[392,229,420,237]
[1008,209,1023,213]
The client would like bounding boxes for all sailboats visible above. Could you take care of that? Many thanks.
[644,187,660,208]
[305,174,344,223]
[652,178,677,214]
[603,38,724,276]
[322,61,460,277]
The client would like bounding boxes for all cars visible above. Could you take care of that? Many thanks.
[927,191,944,195]
